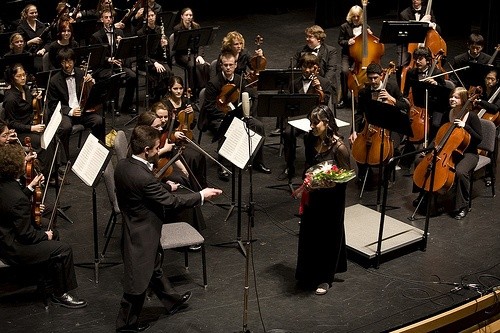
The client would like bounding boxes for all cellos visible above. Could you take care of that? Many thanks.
[348,0,385,103]
[408,95,476,222]
[350,61,398,199]
[477,42,500,158]
[400,0,446,108]
[409,49,445,143]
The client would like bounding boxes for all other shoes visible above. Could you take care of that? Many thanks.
[270,128,286,135]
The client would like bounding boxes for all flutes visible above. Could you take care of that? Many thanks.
[115,38,123,72]
[160,16,168,59]
[120,0,141,24]
[72,0,82,20]
[30,3,71,56]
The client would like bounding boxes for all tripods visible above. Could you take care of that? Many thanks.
[214,116,249,222]
[174,26,213,111]
[208,125,257,257]
[40,100,76,225]
[115,35,147,126]
[71,133,124,283]
[258,69,319,201]
[360,95,415,211]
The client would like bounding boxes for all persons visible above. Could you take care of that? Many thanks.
[348,61,408,186]
[50,2,76,41]
[17,5,52,55]
[215,31,264,79]
[277,54,336,181]
[130,0,161,37]
[0,33,32,57]
[197,51,271,181]
[126,102,208,253]
[403,46,445,143]
[450,34,494,90]
[48,22,79,69]
[113,126,223,333]
[160,75,207,191]
[412,88,483,219]
[395,0,441,89]
[2,64,70,188]
[173,9,212,103]
[90,9,137,117]
[136,9,175,102]
[0,121,88,309]
[335,5,378,109]
[295,106,359,295]
[47,48,107,176]
[76,0,130,22]
[270,25,336,135]
[473,70,500,187]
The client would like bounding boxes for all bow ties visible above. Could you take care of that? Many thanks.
[106,31,113,34]
[226,79,234,84]
[65,73,75,79]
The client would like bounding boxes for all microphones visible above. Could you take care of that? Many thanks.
[242,92,250,118]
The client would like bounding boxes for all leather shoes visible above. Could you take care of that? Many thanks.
[278,167,295,181]
[114,108,121,116]
[116,323,150,333]
[253,163,271,174]
[120,106,136,112]
[455,207,472,219]
[216,168,229,177]
[169,290,193,315]
[46,291,87,308]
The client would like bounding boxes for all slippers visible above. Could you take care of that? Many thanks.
[315,283,329,294]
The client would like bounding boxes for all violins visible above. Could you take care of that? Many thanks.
[175,89,193,142]
[32,80,43,126]
[309,64,323,119]
[24,135,34,188]
[214,73,253,112]
[33,168,41,229]
[81,60,103,114]
[250,35,267,88]
[153,108,178,178]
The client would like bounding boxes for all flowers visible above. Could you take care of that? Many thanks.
[293,160,357,214]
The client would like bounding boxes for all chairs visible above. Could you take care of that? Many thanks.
[0,0,208,310]
[390,79,496,211]
